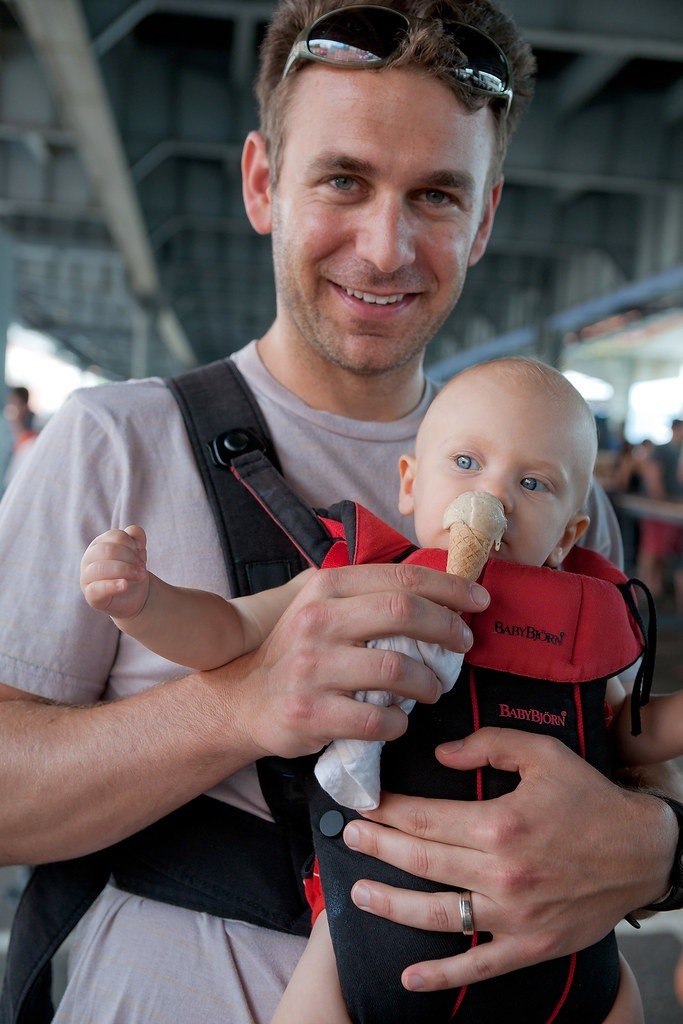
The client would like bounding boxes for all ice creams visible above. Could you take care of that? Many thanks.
[443,489,508,615]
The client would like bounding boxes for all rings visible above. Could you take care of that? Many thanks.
[458,888,477,939]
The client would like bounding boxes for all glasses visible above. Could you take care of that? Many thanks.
[282,5,512,120]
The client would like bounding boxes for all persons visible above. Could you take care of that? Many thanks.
[6,384,683,626]
[2,0,683,1024]
[78,354,683,1024]
